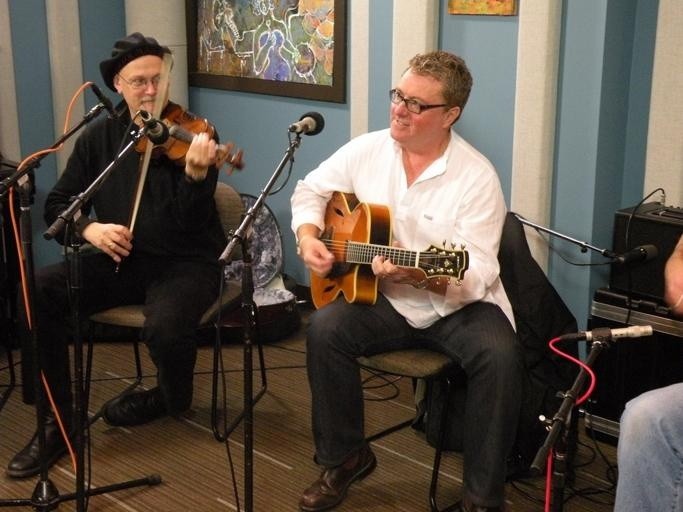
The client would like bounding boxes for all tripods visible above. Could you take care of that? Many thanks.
[0,257,163,512]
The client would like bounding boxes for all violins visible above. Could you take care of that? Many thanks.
[134,102,243,174]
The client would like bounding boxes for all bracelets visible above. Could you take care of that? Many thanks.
[296,235,314,256]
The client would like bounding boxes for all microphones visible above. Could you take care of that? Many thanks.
[287,110,325,138]
[629,240,658,264]
[90,81,124,124]
[582,322,655,342]
[140,108,171,144]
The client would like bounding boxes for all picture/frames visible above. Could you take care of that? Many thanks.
[185,0,345,104]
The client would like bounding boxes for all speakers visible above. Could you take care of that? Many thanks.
[609,200,683,307]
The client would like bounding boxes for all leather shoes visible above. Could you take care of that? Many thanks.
[103,388,164,427]
[7,415,66,478]
[299,445,376,511]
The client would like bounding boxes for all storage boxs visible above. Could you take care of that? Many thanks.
[583,287,683,441]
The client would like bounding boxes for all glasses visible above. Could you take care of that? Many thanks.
[390,90,450,115]
[115,68,162,89]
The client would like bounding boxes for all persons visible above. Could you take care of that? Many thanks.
[613,232,683,511]
[7,34,218,480]
[289,51,525,512]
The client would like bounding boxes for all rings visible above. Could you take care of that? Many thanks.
[108,241,115,249]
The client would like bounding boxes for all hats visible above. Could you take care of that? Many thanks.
[99,32,171,92]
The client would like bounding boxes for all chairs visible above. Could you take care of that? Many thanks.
[356,211,532,511]
[83,180,267,442]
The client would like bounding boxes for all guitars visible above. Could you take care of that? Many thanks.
[309,192,469,310]
